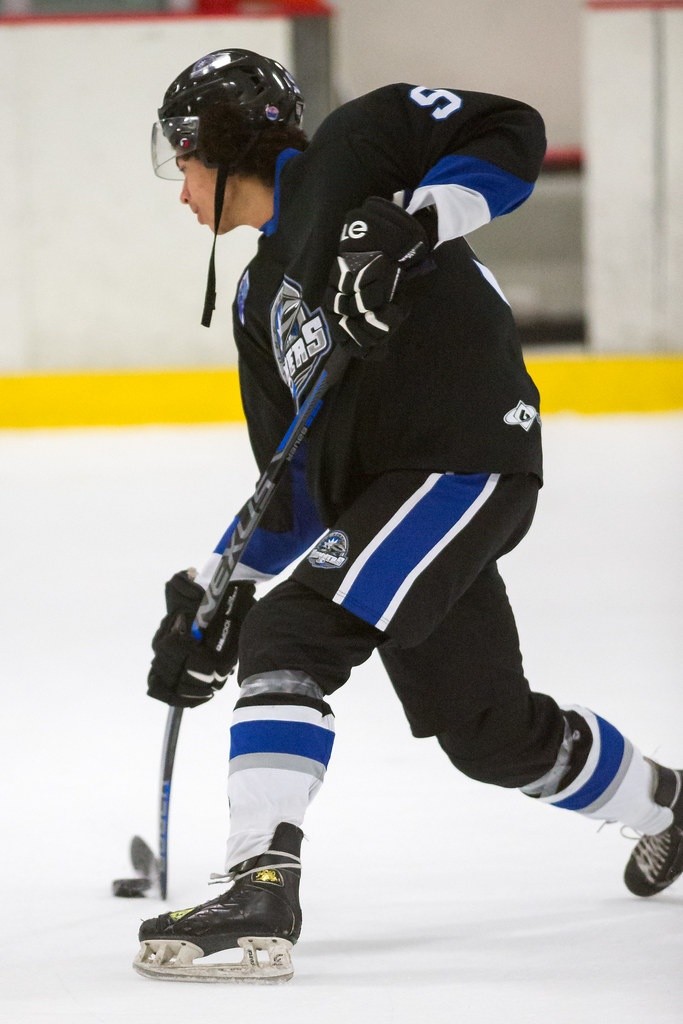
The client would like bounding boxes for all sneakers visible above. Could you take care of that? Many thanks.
[133,822,305,983]
[598,756,683,897]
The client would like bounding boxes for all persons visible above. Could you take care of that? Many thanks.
[135,49,683,985]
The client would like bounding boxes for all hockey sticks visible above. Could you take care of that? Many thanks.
[128,338,359,903]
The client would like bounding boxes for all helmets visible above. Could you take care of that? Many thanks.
[157,49,305,154]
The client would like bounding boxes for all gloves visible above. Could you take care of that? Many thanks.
[147,568,258,708]
[322,195,430,359]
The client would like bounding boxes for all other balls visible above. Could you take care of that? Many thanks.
[113,877,151,897]
